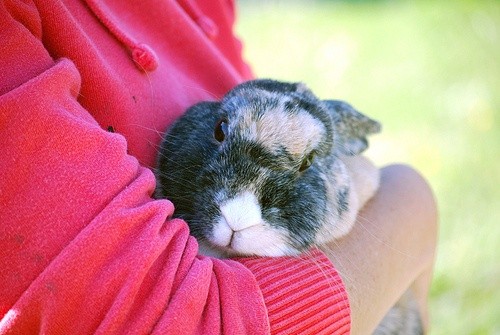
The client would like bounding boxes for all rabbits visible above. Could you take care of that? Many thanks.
[142,78,423,335]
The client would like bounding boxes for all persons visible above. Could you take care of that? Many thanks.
[1,0,438,335]
